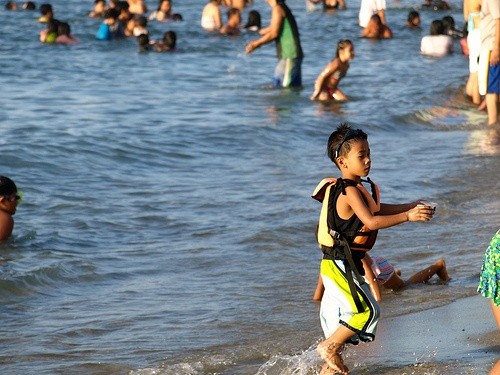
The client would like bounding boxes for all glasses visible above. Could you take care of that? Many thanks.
[5,191,23,203]
[335,129,368,158]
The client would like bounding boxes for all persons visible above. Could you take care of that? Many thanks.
[455,0,499,131]
[311,250,452,303]
[4,0,268,59]
[310,37,355,103]
[398,10,422,34]
[246,0,305,92]
[0,174,21,250]
[476,219,500,375]
[421,19,454,62]
[311,122,437,374]
[438,14,461,42]
[298,1,393,39]
